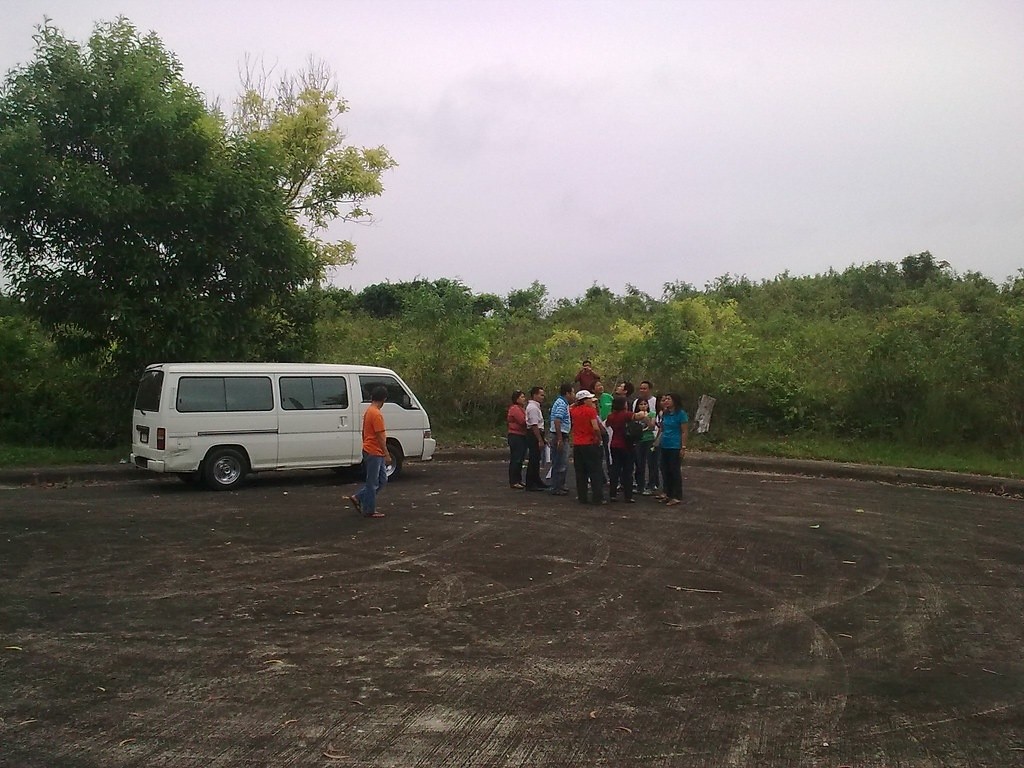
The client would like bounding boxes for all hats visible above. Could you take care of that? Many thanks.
[575,390,596,399]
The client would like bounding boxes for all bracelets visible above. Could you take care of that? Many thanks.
[682,446,687,448]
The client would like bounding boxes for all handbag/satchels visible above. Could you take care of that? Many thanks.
[625,414,644,438]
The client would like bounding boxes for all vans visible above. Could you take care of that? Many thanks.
[129,361,437,491]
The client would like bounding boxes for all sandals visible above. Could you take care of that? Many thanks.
[666,500,681,505]
[659,498,675,503]
[365,511,388,517]
[350,496,362,514]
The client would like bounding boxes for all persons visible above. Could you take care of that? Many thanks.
[508,360,688,506]
[349,385,393,517]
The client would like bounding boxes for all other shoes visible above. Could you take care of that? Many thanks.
[551,491,568,496]
[610,497,619,502]
[510,484,523,489]
[562,487,569,491]
[625,498,638,503]
[525,486,545,491]
[519,482,527,486]
